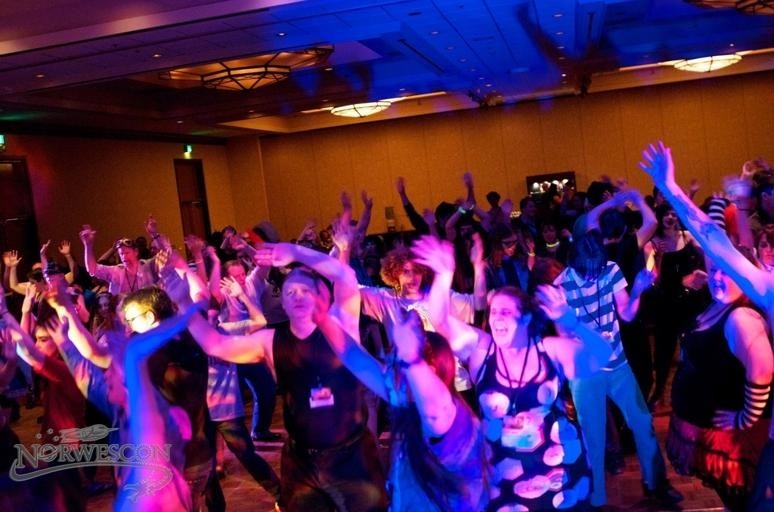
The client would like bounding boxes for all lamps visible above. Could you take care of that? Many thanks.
[158,45,336,92]
[619,48,774,73]
[300,92,447,118]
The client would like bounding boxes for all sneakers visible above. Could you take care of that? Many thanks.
[378,432,392,448]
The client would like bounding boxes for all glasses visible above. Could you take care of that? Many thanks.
[115,239,133,248]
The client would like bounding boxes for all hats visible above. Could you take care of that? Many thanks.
[45,261,68,274]
[435,202,459,220]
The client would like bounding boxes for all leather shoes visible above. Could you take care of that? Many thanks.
[644,483,683,503]
[648,398,664,410]
[250,430,282,440]
[608,450,626,474]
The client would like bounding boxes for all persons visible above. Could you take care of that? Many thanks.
[1,142,773,512]
